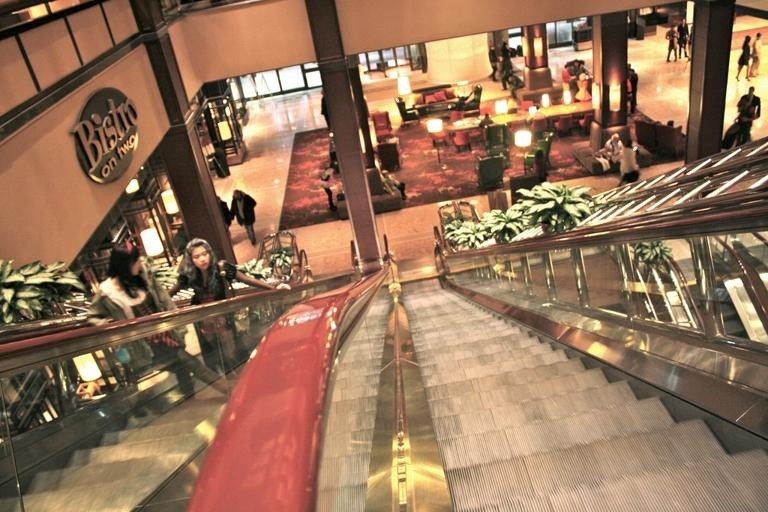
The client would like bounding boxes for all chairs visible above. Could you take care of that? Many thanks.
[523,137,550,182]
[412,82,595,163]
[395,96,420,126]
[371,111,394,143]
[634,116,686,159]
[474,152,505,193]
[561,64,593,91]
[377,142,401,171]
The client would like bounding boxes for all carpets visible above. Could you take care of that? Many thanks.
[277,94,687,231]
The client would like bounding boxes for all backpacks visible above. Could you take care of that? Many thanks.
[195,288,229,336]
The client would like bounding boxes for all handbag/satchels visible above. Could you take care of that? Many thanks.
[231,306,252,339]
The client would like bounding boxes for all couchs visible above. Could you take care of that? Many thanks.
[571,121,633,176]
[513,67,563,102]
